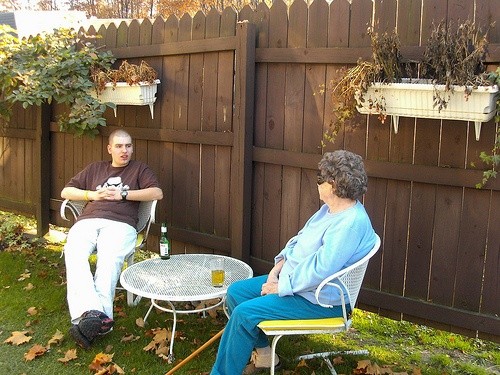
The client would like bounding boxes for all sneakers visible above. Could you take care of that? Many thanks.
[79,310,114,337]
[68,326,92,348]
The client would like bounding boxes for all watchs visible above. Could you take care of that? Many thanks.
[120,190,128,201]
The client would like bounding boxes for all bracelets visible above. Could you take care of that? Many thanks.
[84,190,89,200]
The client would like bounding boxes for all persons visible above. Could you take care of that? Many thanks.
[208,151,375,375]
[57,129,163,347]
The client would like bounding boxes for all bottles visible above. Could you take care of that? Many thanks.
[159,223,171,260]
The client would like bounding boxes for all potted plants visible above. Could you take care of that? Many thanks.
[84,59,160,119]
[319,17,500,189]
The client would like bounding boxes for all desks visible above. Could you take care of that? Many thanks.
[119,253,253,364]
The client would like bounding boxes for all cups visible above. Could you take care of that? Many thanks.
[208,257,225,287]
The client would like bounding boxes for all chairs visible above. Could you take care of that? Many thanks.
[255,232,382,375]
[61,199,157,306]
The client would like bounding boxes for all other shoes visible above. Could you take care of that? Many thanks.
[245,354,282,375]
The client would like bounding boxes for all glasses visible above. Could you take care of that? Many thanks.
[317,174,332,185]
